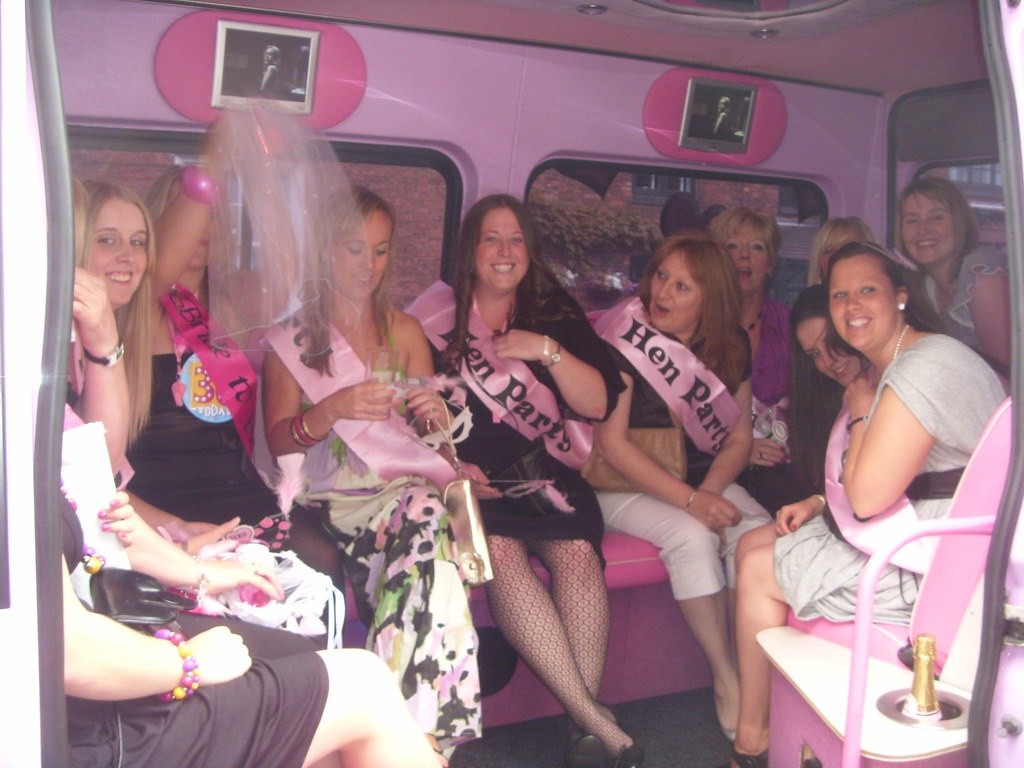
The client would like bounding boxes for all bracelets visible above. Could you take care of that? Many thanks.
[537,333,549,363]
[686,488,699,509]
[153,629,199,703]
[173,553,210,598]
[179,162,226,204]
[846,416,868,431]
[288,409,329,448]
[810,494,826,505]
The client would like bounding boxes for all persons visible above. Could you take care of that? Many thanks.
[261,46,283,101]
[581,206,793,745]
[63,108,443,768]
[260,181,648,768]
[711,96,731,140]
[726,177,1011,768]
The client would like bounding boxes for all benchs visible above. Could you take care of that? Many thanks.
[784,399,1014,681]
[343,529,716,729]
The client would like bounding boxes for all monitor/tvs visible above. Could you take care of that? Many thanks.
[211,19,320,115]
[677,76,758,153]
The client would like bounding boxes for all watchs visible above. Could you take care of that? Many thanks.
[544,341,561,369]
[82,336,124,367]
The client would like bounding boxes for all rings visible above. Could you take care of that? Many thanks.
[759,452,764,458]
[768,408,771,413]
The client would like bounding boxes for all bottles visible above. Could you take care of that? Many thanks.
[901,635,943,722]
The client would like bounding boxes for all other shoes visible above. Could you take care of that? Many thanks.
[730,746,771,768]
[723,723,738,743]
[565,725,645,768]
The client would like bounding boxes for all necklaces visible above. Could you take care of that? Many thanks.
[892,323,910,359]
[745,312,762,332]
[61,479,106,574]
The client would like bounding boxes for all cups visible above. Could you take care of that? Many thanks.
[364,345,399,419]
[753,404,793,463]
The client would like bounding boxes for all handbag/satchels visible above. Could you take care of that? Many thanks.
[91,568,193,633]
[580,421,686,495]
